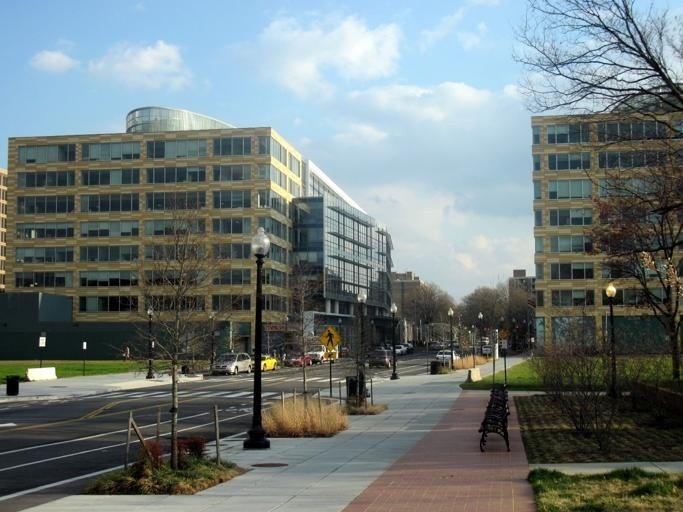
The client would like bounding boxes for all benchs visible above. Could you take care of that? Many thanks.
[477,381,512,452]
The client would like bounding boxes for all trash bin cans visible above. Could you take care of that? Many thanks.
[346,376,358,398]
[182,366,189,374]
[430,361,440,374]
[6,375,20,396]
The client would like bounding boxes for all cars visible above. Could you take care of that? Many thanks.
[208,343,339,376]
[368,335,497,369]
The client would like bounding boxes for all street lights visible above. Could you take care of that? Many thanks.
[354,288,367,402]
[242,225,272,449]
[447,306,455,371]
[498,314,526,356]
[605,282,617,417]
[477,312,483,357]
[389,301,399,379]
[146,306,156,380]
